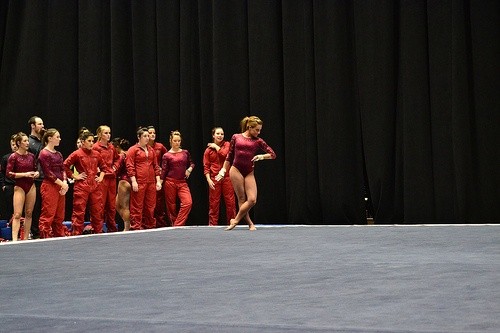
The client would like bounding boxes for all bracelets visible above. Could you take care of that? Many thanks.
[187,170,191,173]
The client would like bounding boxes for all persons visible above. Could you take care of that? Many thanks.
[160,130,195,226]
[146,126,168,227]
[28,116,46,239]
[111,138,131,230]
[127,128,162,229]
[92,125,125,232]
[66,137,82,184]
[63,126,109,236]
[0,134,18,228]
[38,129,69,239]
[214,116,276,230]
[204,127,237,226]
[6,132,39,242]
